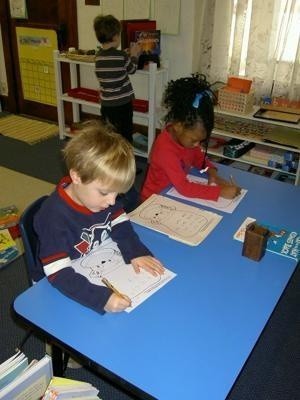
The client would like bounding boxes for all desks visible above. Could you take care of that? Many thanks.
[11,160,300,399]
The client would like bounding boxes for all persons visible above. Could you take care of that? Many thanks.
[139,76,241,202]
[28,119,165,370]
[93,15,138,145]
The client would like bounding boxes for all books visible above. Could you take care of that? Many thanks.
[0,349,103,400]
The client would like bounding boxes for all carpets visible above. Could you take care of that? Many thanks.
[1,114,59,145]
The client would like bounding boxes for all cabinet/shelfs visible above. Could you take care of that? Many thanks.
[201,109,299,186]
[50,45,170,164]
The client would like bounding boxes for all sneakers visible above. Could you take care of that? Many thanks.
[42,340,82,370]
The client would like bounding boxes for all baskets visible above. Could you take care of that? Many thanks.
[216,83,254,116]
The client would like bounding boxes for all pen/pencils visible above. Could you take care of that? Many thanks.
[229,175,237,186]
[101,278,133,308]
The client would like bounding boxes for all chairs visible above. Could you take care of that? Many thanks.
[17,195,69,377]
